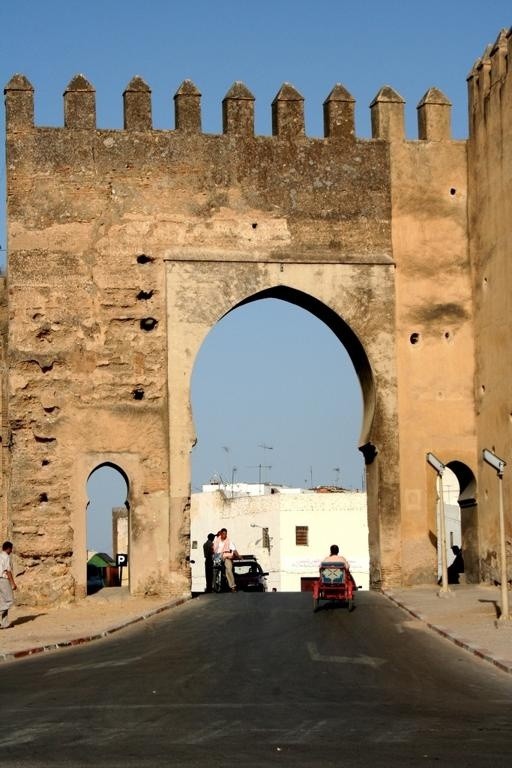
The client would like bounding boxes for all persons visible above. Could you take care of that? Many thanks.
[272,588,277,593]
[212,528,244,592]
[323,545,349,571]
[0,541,18,628]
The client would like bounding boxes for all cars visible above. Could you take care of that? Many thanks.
[221,552,269,593]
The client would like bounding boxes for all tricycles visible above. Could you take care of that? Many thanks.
[311,563,356,614]
